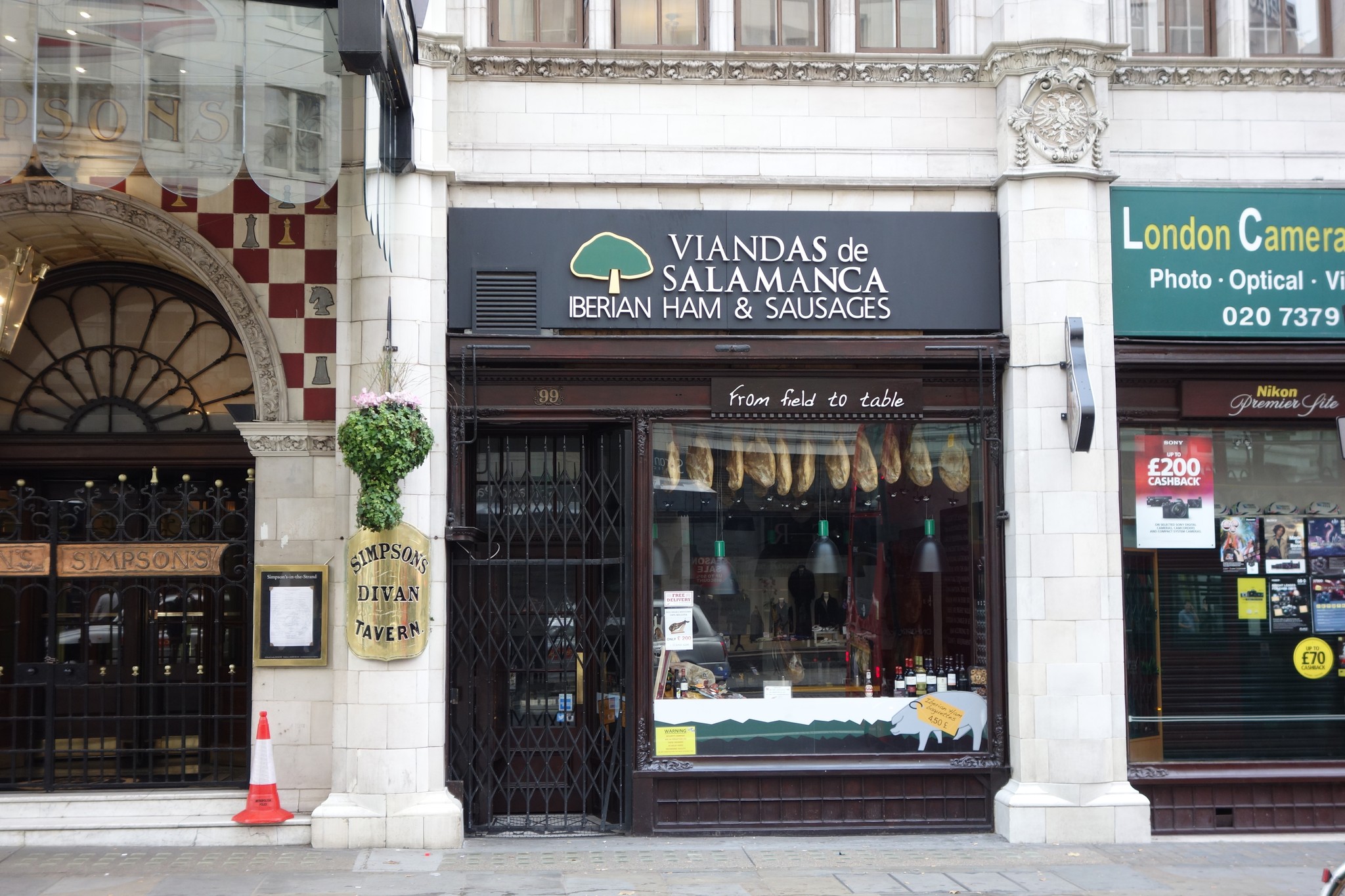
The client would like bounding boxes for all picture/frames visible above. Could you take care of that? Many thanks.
[251,564,329,667]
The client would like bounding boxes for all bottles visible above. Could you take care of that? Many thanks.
[865,654,970,699]
[673,668,687,699]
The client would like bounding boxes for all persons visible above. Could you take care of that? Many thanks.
[1241,538,1255,560]
[42,578,236,671]
[1200,599,1217,631]
[691,556,852,653]
[1265,524,1289,561]
[1177,599,1200,635]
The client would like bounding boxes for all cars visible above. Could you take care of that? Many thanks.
[45,589,235,665]
[540,601,733,686]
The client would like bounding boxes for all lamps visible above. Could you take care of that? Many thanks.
[704,422,738,596]
[651,505,668,576]
[808,454,843,574]
[911,483,949,572]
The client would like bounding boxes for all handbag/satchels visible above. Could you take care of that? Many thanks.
[1266,546,1281,559]
